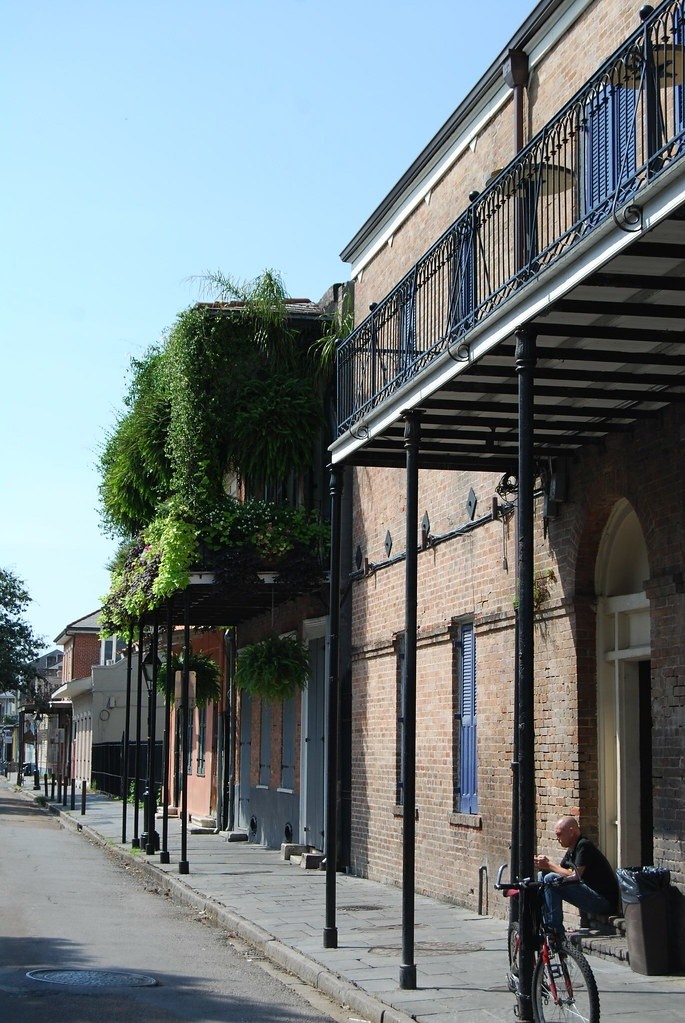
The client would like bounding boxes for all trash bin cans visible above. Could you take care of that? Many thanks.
[616,865,670,976]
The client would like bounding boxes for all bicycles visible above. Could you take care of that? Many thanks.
[493,861,601,1023]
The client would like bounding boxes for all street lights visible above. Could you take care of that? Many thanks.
[32,712,43,790]
[142,644,161,851]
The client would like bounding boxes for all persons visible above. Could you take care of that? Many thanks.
[534,815,620,935]
